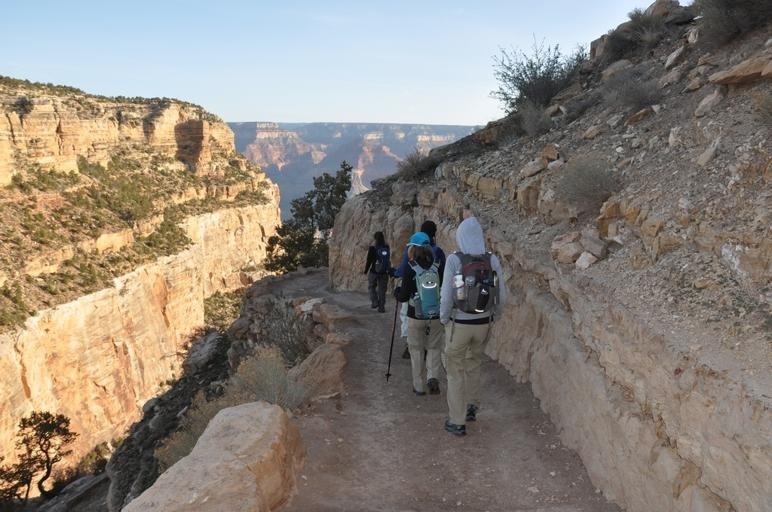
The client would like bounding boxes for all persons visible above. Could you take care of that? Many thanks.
[438,216,509,436]
[393,231,447,395]
[363,231,391,314]
[391,220,447,360]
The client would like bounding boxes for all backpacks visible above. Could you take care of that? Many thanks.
[408,259,442,318]
[452,250,500,314]
[371,246,391,274]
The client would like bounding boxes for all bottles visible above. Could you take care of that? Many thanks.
[413,292,422,320]
[452,269,476,302]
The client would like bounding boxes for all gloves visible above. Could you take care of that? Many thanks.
[390,268,396,276]
[394,287,400,297]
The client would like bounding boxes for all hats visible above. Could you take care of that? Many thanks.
[407,232,430,246]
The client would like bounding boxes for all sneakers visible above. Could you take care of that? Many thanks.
[403,347,410,358]
[446,420,466,435]
[428,378,440,395]
[412,387,424,394]
[371,302,385,313]
[466,408,476,421]
